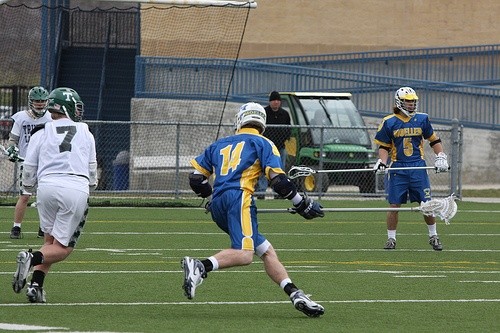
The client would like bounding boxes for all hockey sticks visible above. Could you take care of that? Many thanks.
[257,194,459,225]
[287,163,451,182]
[0,142,25,163]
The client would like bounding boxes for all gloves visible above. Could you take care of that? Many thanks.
[6,146,19,162]
[373,159,388,175]
[434,152,448,174]
[293,195,324,220]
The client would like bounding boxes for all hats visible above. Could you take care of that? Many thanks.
[269,91,281,101]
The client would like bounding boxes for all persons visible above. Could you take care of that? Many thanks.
[3,86,56,239]
[255,91,292,199]
[12,87,99,303]
[373,87,450,251]
[308,107,331,141]
[180,102,325,317]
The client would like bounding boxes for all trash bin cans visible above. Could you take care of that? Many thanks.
[111,150,130,190]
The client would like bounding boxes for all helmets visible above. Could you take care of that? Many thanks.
[235,102,267,134]
[46,87,84,119]
[395,87,419,118]
[28,86,49,118]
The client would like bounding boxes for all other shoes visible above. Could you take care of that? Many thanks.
[257,195,265,199]
[274,195,286,199]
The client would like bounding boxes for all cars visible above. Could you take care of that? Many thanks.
[0,105,31,140]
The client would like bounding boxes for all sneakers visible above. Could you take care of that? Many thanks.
[290,289,325,318]
[180,256,208,299]
[384,238,396,248]
[12,248,35,294]
[428,235,443,251]
[26,279,47,303]
[10,226,23,239]
[37,229,45,238]
